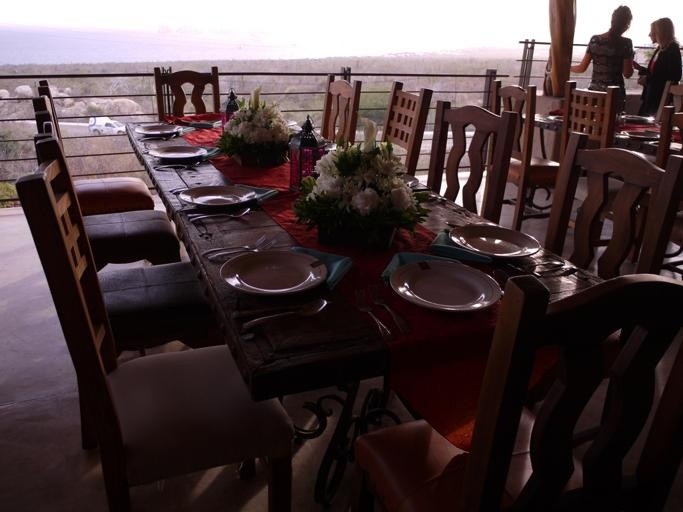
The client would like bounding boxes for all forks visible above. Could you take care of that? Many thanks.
[352,288,391,338]
[508,262,564,271]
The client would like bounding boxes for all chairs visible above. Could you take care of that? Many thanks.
[424,100,518,225]
[319,74,366,147]
[38,80,157,213]
[479,67,683,272]
[380,82,432,177]
[30,95,183,273]
[16,137,303,509]
[545,131,683,281]
[335,270,683,511]
[26,121,226,448]
[153,66,219,117]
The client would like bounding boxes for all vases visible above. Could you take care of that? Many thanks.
[237,140,284,169]
[350,220,400,253]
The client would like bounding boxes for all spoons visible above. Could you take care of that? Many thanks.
[243,299,328,329]
[369,283,403,332]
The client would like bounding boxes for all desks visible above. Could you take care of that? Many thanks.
[124,120,609,512]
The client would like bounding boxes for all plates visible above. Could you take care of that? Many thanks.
[136,124,327,294]
[400,174,418,187]
[390,223,541,311]
[623,130,683,152]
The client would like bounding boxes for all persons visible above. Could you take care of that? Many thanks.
[570,5,636,119]
[633,17,683,117]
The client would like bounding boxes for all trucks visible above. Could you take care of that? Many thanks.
[88,117,126,135]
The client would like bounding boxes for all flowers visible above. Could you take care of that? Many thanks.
[218,105,297,142]
[310,142,424,219]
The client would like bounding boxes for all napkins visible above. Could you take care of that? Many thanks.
[197,145,219,161]
[428,231,493,265]
